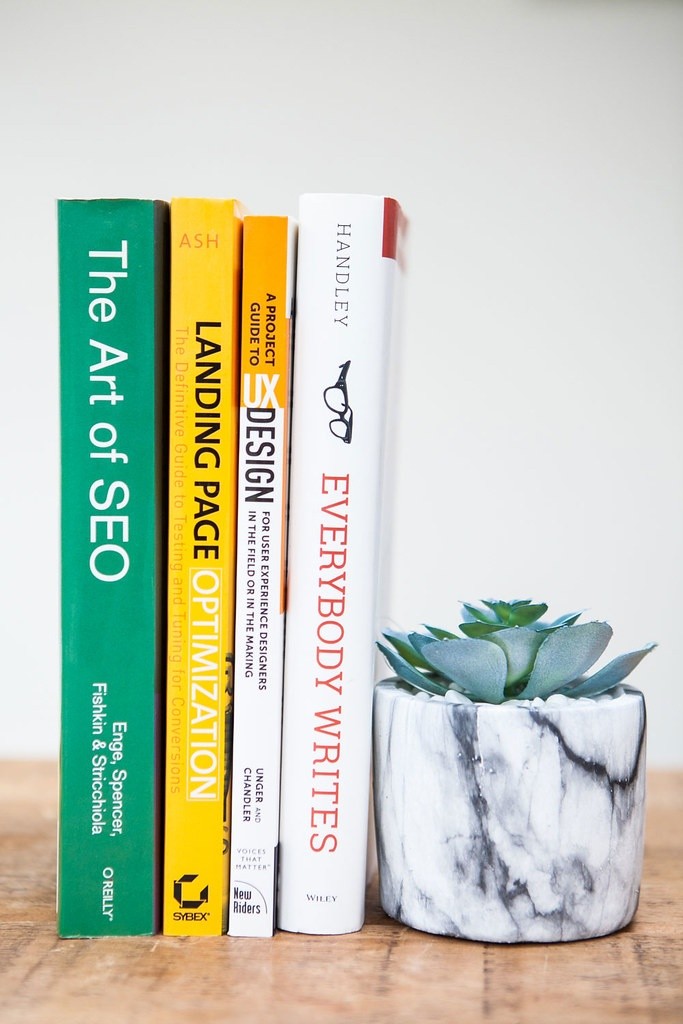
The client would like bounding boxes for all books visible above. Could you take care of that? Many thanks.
[279,193,407,935]
[161,200,243,937]
[57,198,171,942]
[228,216,299,939]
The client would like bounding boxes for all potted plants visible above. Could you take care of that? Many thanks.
[375,598,659,942]
